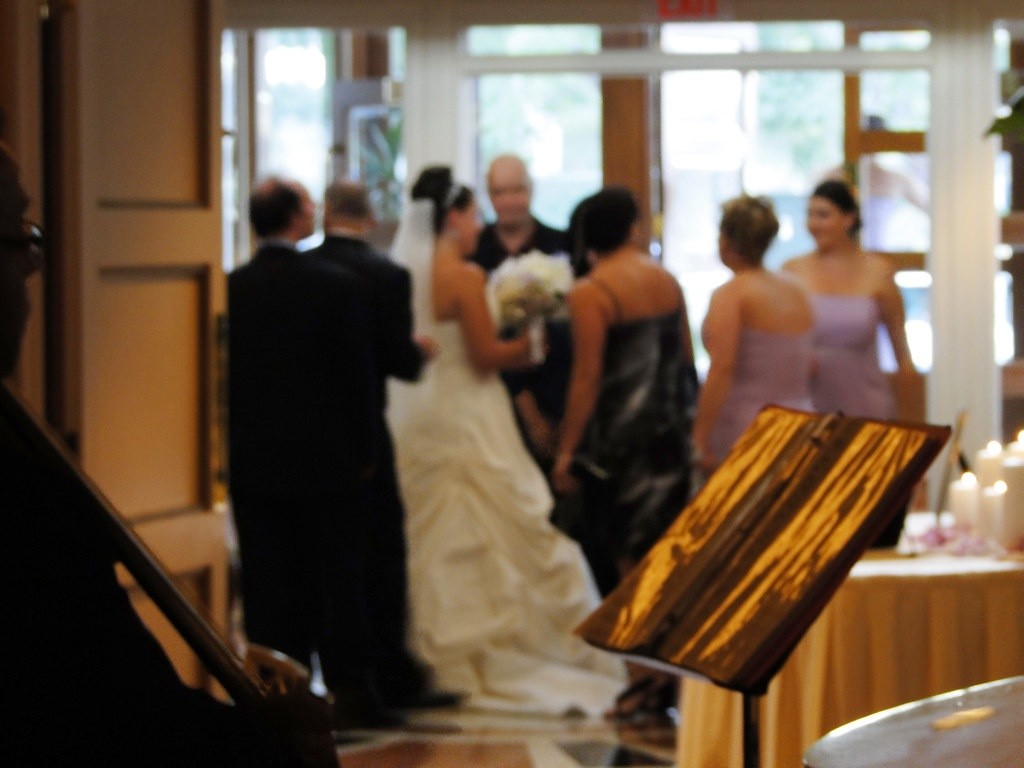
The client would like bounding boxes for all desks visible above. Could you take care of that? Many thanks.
[677,549,1024,768]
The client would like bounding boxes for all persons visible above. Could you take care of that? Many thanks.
[225,154,919,715]
[0,150,339,768]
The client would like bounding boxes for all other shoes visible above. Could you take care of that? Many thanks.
[393,679,466,709]
[605,674,677,722]
[335,704,406,729]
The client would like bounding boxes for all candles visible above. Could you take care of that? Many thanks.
[950,429,1023,551]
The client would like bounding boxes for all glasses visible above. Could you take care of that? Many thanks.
[0,221,48,278]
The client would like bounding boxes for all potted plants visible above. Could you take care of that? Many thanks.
[358,118,404,222]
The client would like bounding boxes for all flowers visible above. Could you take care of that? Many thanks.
[829,162,860,199]
[485,250,574,333]
